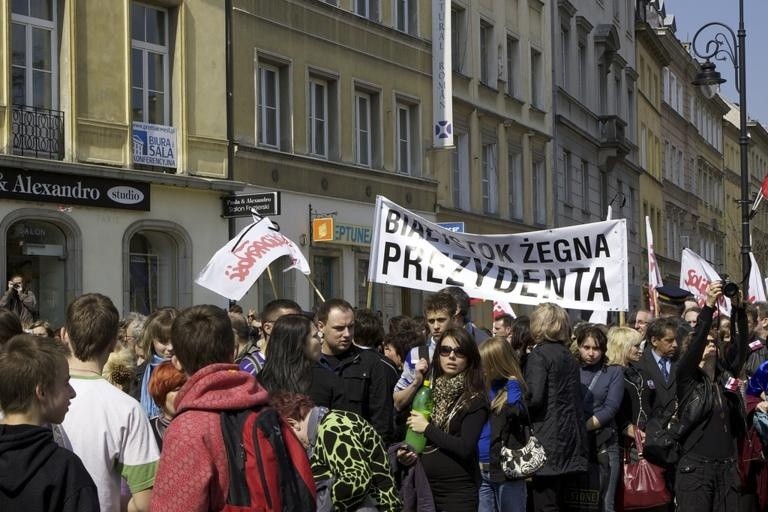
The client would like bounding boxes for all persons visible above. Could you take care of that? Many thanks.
[0,274,41,332]
[0,278,766,511]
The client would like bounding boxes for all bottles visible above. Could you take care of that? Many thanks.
[403,380,434,454]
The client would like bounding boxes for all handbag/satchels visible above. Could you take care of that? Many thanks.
[644,414,680,466]
[499,436,548,480]
[623,458,672,510]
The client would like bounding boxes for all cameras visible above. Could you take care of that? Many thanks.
[717,280,739,299]
[13,282,21,288]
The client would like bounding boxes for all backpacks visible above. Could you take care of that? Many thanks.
[219,403,318,511]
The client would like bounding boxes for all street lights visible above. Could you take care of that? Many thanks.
[693,16,757,314]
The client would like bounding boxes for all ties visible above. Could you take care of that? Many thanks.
[659,357,669,385]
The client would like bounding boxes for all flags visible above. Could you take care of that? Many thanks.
[679,246,732,316]
[644,214,664,318]
[745,248,767,305]
[193,217,327,304]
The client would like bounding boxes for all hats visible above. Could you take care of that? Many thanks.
[656,286,694,305]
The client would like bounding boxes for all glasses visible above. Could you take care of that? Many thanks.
[439,346,465,358]
